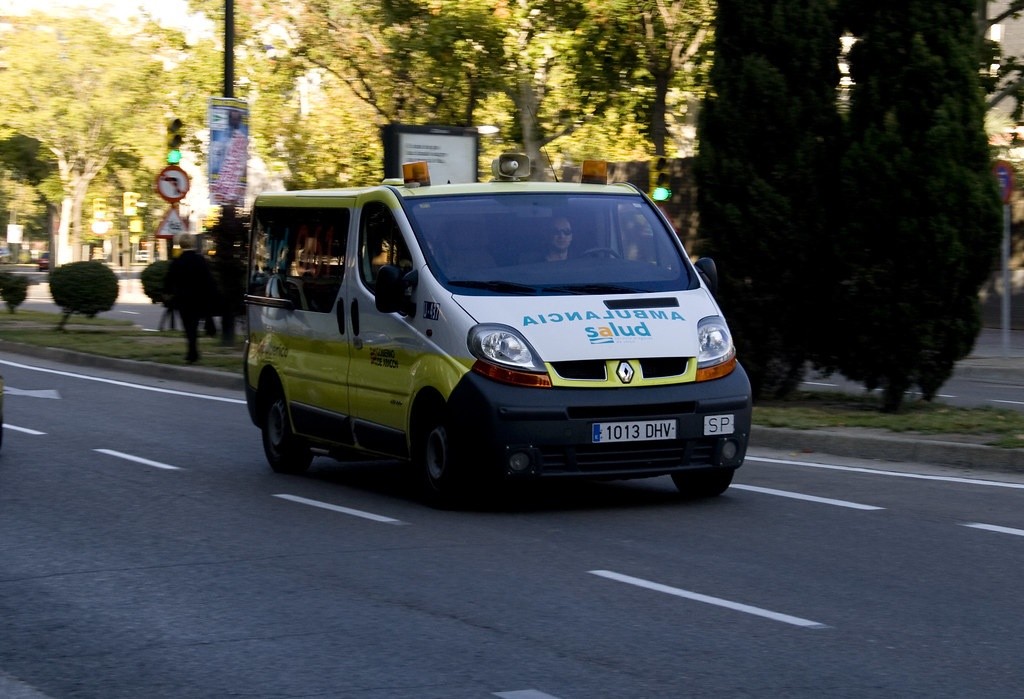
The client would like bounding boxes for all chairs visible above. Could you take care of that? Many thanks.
[440,214,499,273]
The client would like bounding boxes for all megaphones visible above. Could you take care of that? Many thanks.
[492,154,530,177]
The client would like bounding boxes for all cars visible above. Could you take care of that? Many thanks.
[135,250,149,262]
[35,251,51,270]
[139,238,159,250]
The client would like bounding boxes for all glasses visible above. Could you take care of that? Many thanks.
[550,228,572,235]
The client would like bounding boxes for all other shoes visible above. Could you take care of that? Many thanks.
[182,355,199,366]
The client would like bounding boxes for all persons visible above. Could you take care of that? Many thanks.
[546,216,574,263]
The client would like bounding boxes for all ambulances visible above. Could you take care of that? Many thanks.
[242,182,752,508]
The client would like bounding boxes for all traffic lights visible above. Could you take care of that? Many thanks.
[649,154,673,205]
[167,118,183,165]
